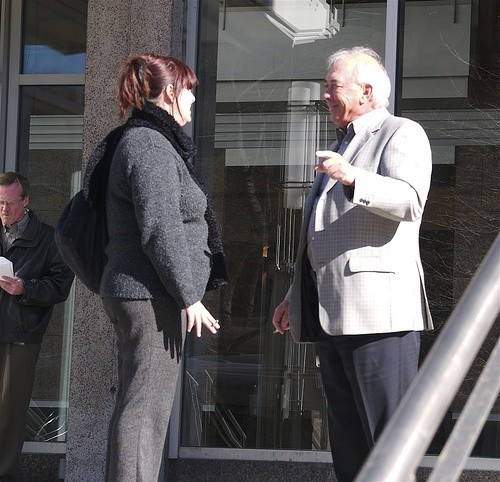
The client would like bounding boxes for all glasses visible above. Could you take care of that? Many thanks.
[0,195,25,209]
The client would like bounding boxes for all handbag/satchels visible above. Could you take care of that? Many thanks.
[54,191,109,294]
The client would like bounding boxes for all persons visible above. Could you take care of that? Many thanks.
[271,45,435,482]
[0,172,75,482]
[99,53,230,482]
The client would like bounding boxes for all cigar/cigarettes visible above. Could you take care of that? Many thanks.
[274,327,290,334]
[211,319,219,327]
